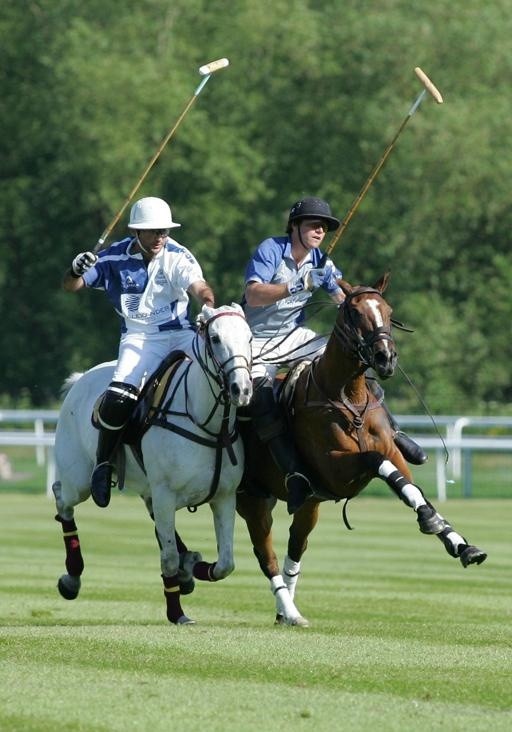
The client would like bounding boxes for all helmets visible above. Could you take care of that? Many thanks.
[127,197,181,232]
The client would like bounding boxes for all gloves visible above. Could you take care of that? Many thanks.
[286,263,326,297]
[70,251,99,278]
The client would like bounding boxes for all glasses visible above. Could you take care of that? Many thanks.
[143,229,172,237]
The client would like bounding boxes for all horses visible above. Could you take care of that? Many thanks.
[48,299,261,628]
[233,270,489,630]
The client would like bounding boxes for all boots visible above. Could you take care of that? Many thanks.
[374,388,428,466]
[90,381,140,507]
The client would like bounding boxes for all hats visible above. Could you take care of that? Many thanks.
[289,196,341,232]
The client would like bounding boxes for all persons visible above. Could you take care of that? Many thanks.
[63,197,216,508]
[240,198,430,514]
